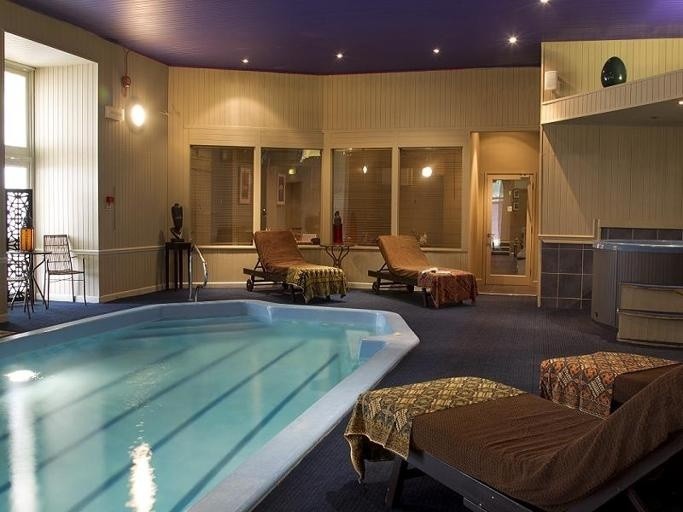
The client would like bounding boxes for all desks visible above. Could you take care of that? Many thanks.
[6,250,51,312]
[321,244,354,270]
[166,241,194,293]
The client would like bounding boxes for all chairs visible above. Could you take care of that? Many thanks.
[43,235,88,308]
[368,235,479,309]
[6,277,30,320]
[344,377,683,512]
[243,231,346,304]
[538,352,683,420]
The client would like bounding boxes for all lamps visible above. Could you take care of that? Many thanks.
[288,168,296,174]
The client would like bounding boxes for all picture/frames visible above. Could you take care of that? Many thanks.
[238,167,252,205]
[277,174,286,205]
